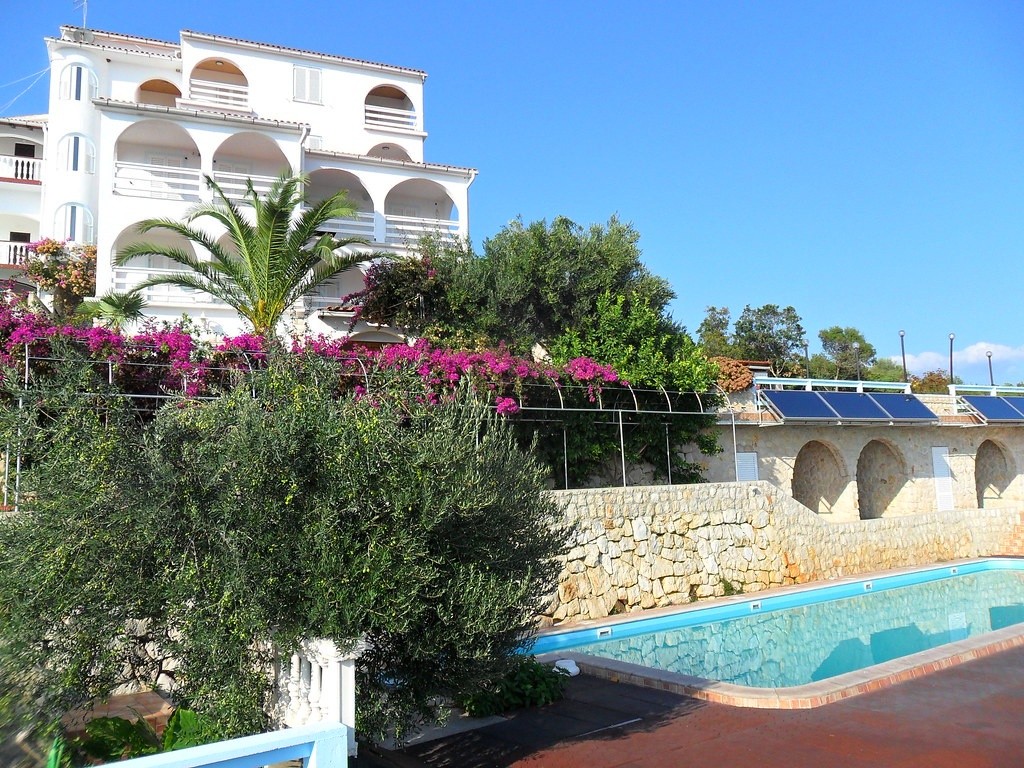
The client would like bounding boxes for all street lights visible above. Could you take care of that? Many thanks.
[852,342,860,381]
[802,338,810,379]
[898,329,908,382]
[948,332,955,384]
[985,350,994,386]
